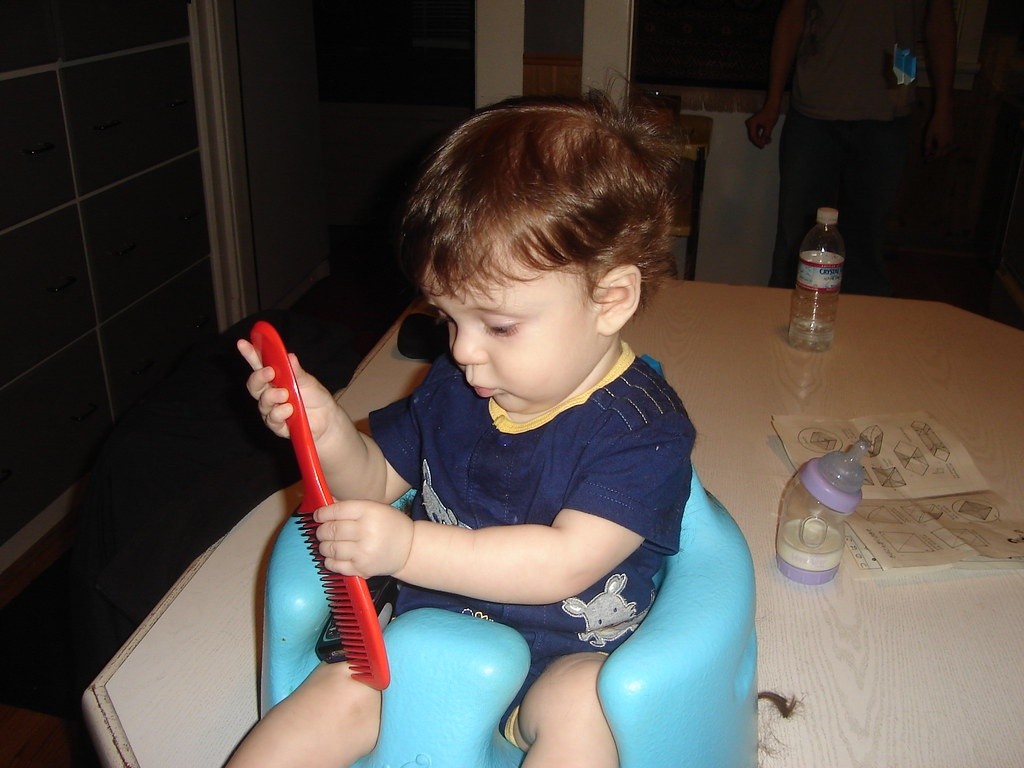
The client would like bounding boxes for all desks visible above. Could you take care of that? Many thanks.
[81,279,1024,768]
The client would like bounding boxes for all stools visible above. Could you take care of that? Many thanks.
[259,467,759,768]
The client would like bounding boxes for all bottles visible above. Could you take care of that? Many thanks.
[787,208,845,352]
[775,440,870,585]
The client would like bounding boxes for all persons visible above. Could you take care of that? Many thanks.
[221,86,694,767]
[745,1,958,302]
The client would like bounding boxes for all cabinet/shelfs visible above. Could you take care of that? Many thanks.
[0,0,219,611]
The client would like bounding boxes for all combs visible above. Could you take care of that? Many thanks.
[248,320,392,692]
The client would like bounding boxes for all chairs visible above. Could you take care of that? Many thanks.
[80,312,360,768]
[634,107,713,280]
